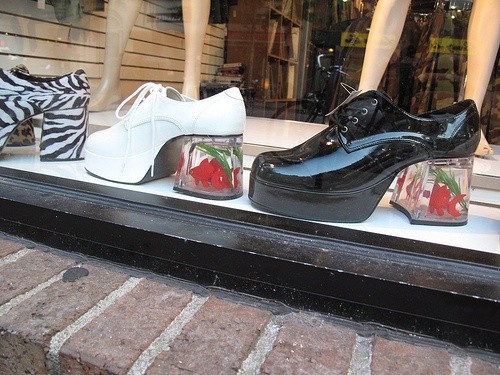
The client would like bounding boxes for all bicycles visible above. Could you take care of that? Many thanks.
[265,51,349,124]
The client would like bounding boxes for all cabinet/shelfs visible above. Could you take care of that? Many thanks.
[226,0,304,121]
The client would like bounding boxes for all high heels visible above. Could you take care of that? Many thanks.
[249,88,481,227]
[84,82,246,200]
[0,63,91,162]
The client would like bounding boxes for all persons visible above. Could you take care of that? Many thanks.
[88,0,210,112]
[357,0,500,155]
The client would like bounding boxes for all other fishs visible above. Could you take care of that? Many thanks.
[176,146,243,197]
[394,168,472,221]
